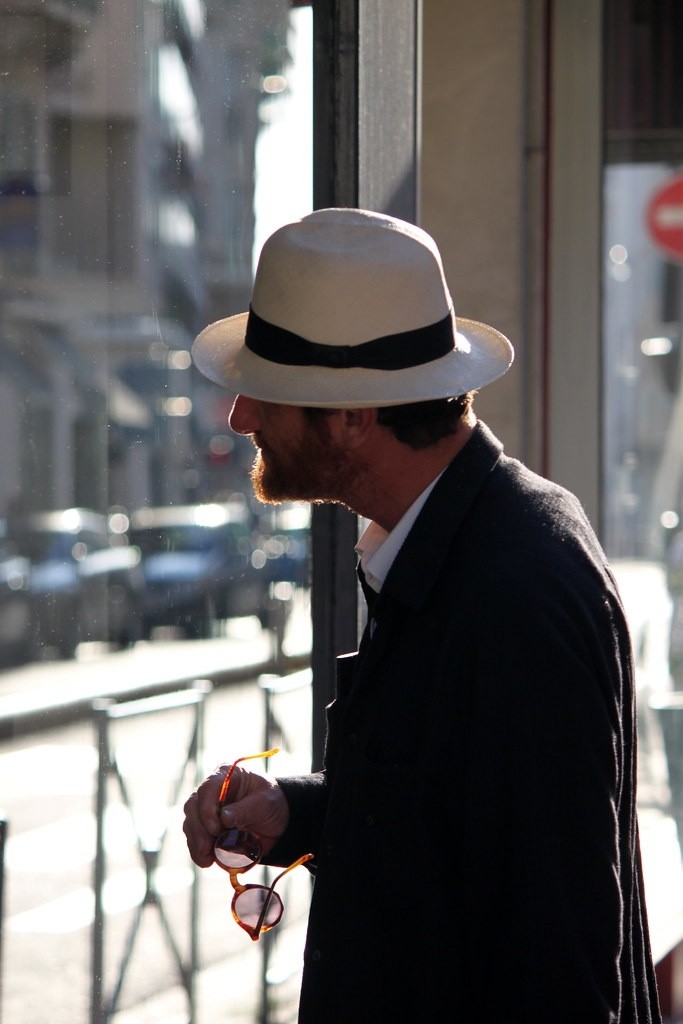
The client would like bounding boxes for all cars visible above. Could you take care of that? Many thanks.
[0,504,311,666]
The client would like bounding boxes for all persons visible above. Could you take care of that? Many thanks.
[182,208,639,1024]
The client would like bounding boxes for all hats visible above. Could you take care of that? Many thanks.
[190,206,514,408]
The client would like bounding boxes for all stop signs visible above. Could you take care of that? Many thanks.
[642,175,683,263]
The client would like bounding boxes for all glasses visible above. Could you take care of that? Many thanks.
[210,747,313,941]
